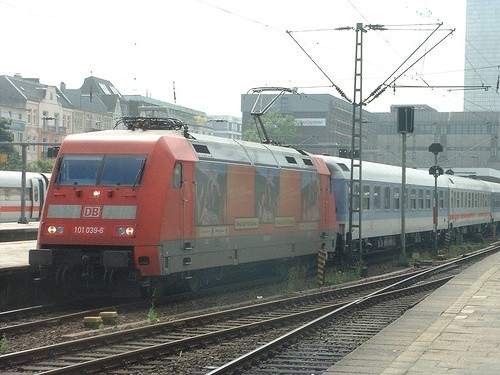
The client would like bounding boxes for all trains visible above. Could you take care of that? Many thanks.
[0,171,53,222]
[28,87,500,305]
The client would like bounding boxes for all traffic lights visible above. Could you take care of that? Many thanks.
[339,149,347,157]
